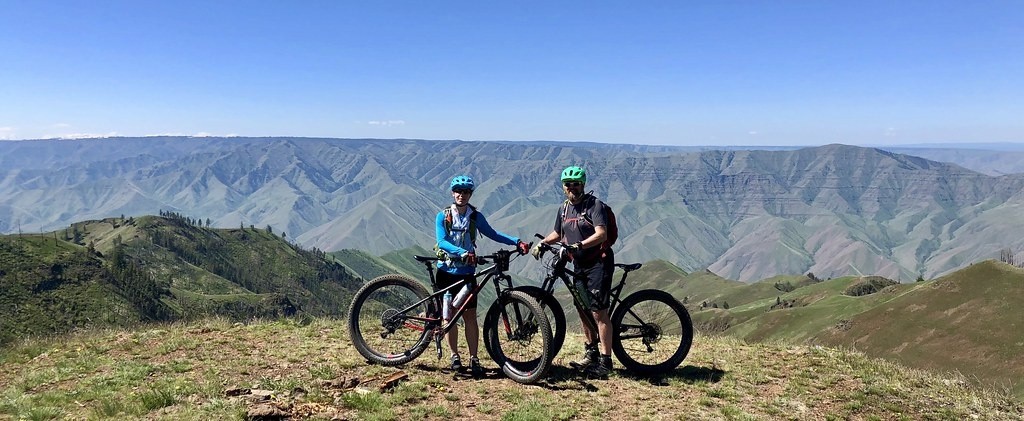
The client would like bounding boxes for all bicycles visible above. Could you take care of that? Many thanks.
[348,241,554,384]
[483,233,693,375]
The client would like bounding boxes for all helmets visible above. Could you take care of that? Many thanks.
[560,166,586,184]
[450,176,475,191]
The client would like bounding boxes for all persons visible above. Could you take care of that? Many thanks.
[435,176,530,377]
[531,166,615,381]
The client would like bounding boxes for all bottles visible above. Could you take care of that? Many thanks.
[451,282,475,308]
[443,290,452,321]
[575,277,592,309]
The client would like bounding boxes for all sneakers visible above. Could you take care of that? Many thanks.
[584,357,612,380]
[569,351,599,371]
[451,354,462,370]
[469,357,483,373]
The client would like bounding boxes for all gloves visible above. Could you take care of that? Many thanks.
[516,241,529,256]
[462,252,477,265]
[559,242,582,260]
[531,241,546,260]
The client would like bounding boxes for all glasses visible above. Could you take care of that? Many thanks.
[564,182,582,187]
[455,189,470,194]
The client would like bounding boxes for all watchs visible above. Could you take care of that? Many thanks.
[575,241,583,251]
